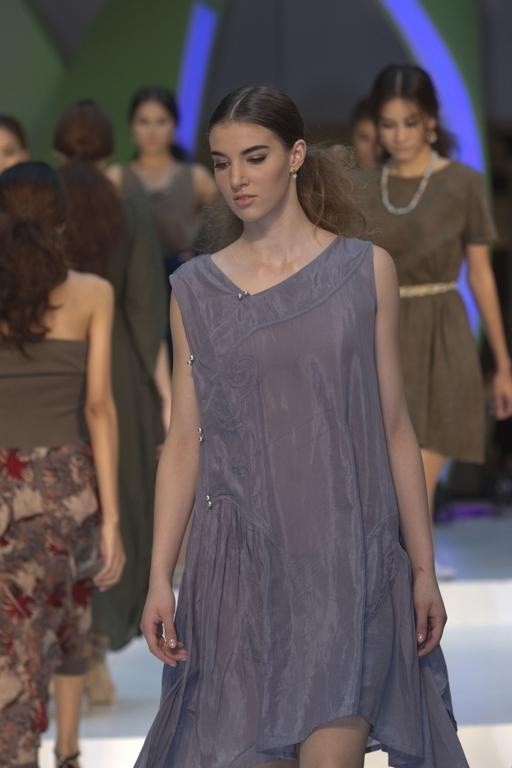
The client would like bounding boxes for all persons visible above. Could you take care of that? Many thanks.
[0,158,129,768]
[351,98,383,170]
[49,99,173,713]
[348,63,511,558]
[129,85,472,768]
[0,114,31,179]
[121,89,217,270]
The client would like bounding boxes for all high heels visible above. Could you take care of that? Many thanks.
[85,655,116,707]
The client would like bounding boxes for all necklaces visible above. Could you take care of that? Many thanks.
[375,150,440,216]
